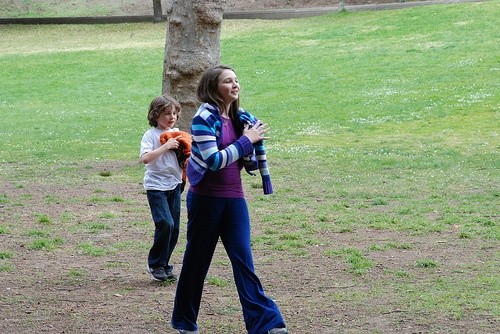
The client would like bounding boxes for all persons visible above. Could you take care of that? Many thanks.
[140,95,183,283]
[171,65,287,334]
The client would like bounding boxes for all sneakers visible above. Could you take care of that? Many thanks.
[165,266,177,283]
[147,264,167,281]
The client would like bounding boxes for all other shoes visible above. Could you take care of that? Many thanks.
[267,326,288,334]
[171,324,199,334]
